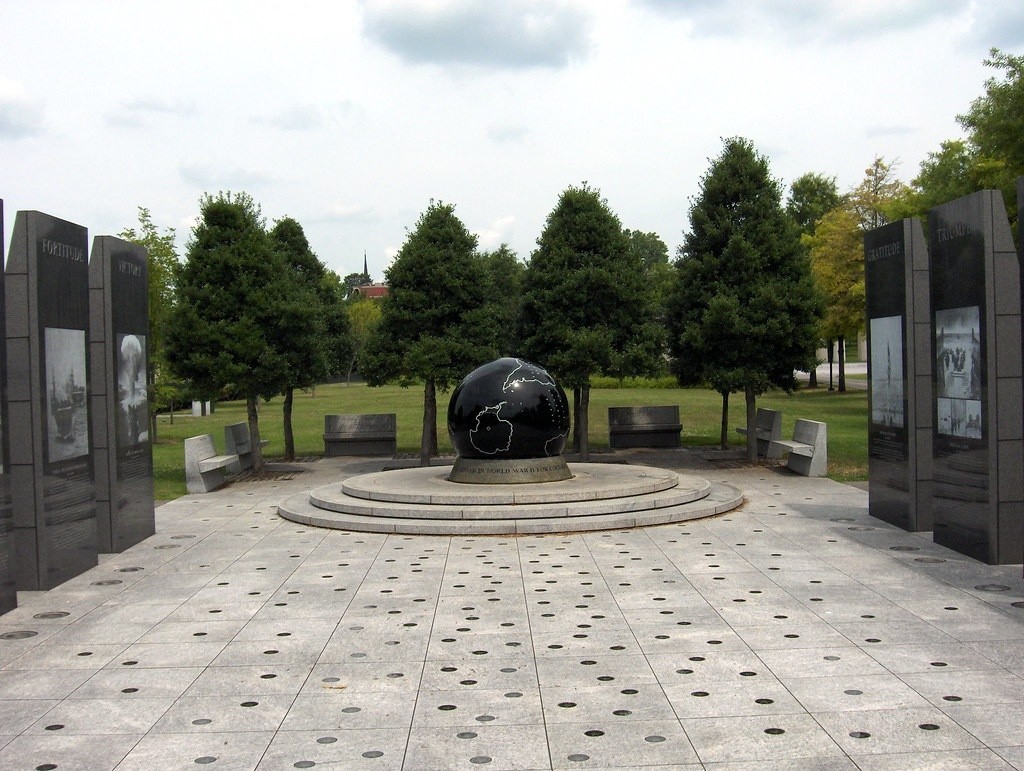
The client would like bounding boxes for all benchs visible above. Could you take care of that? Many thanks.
[771,418,828,478]
[184,432,240,495]
[322,413,397,456]
[735,407,784,459]
[225,421,268,474]
[608,405,683,448]
